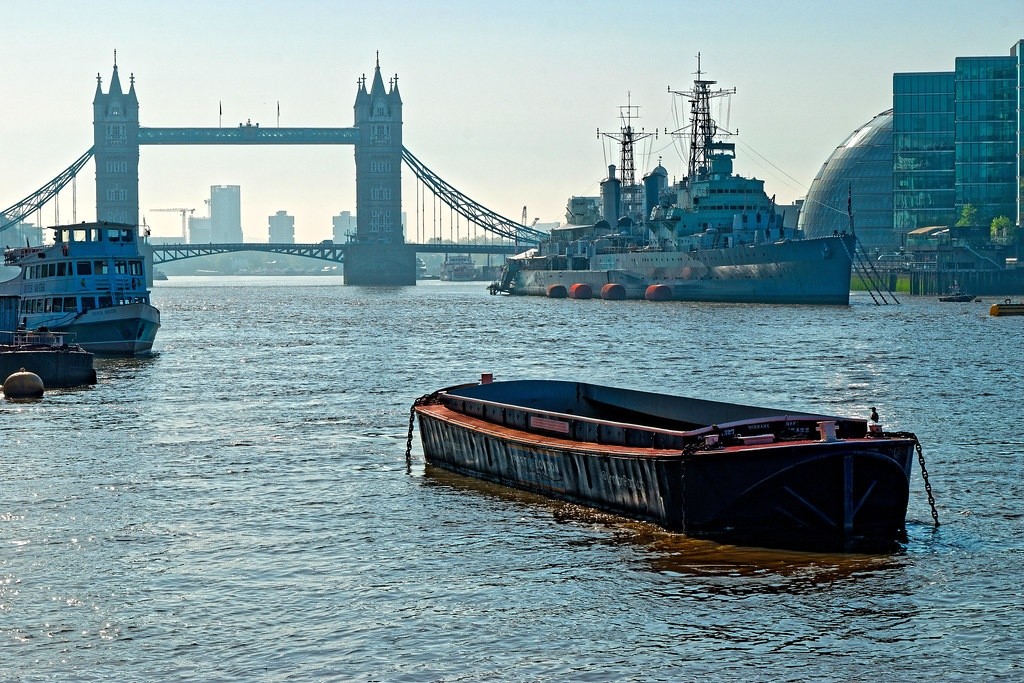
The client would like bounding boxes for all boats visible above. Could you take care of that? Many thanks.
[0,222,162,357]
[938,290,976,304]
[406,378,939,542]
[0,324,97,392]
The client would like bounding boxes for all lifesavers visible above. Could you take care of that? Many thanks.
[1004,298,1011,304]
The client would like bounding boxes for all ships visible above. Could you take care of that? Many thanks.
[486,153,858,307]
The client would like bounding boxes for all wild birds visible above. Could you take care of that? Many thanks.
[869,407,879,425]
